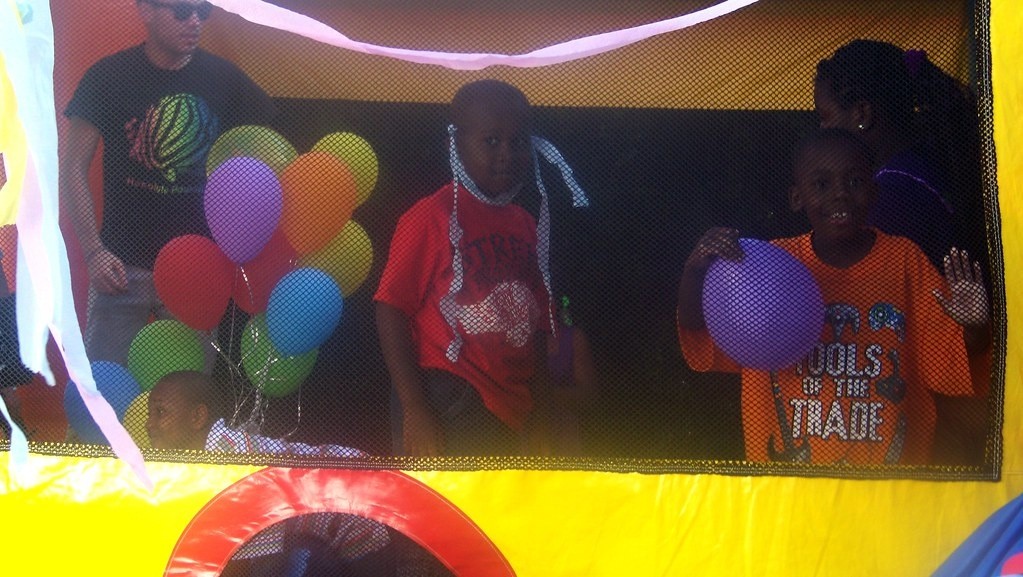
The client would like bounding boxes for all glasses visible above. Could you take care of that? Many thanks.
[150,0,212,20]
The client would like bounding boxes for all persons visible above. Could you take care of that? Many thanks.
[58,0,283,446]
[680,38,992,465]
[145,370,370,457]
[373,80,601,457]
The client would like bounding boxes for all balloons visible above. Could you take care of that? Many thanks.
[699,237,824,369]
[63,119,372,445]
